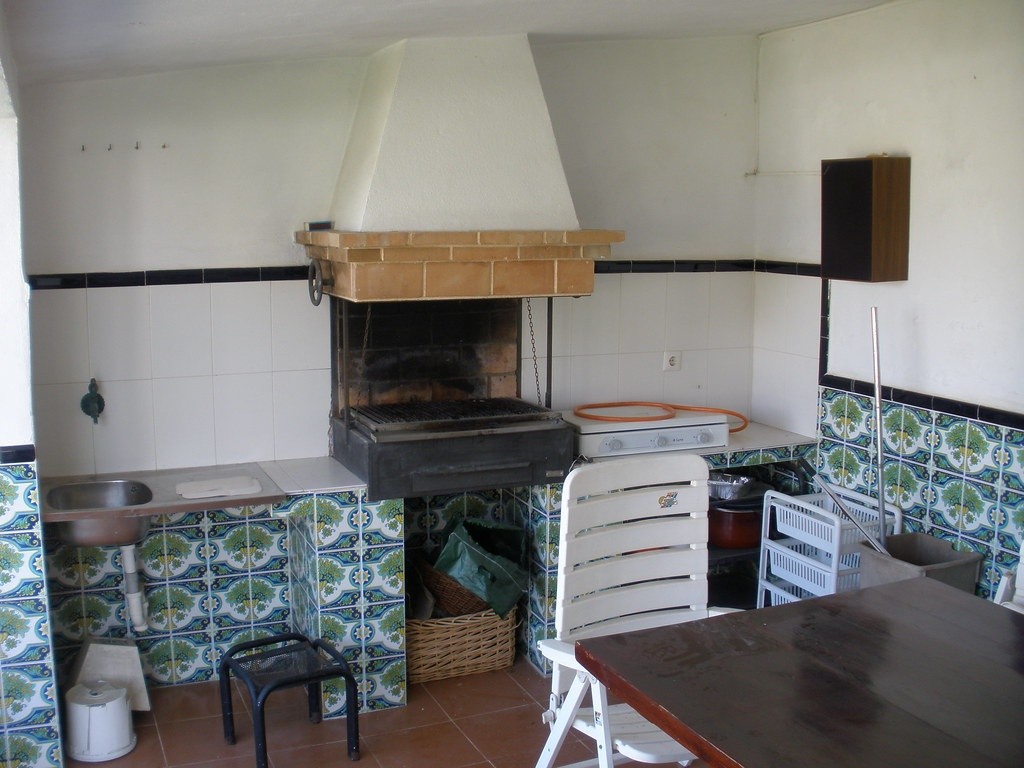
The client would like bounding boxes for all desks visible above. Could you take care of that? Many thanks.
[575,576,1024,768]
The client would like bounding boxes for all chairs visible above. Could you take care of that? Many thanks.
[537,454,748,768]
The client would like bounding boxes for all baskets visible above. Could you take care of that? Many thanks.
[406,605,518,684]
[414,559,491,616]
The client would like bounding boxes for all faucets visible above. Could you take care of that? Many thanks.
[80,378,105,425]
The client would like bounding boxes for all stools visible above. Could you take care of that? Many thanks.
[214,631,362,768]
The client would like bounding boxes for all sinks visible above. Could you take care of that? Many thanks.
[47,480,153,547]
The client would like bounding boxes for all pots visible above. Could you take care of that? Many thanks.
[709,484,777,548]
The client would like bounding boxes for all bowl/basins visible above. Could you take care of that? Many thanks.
[707,471,753,500]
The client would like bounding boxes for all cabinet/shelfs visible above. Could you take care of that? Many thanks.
[756,482,903,609]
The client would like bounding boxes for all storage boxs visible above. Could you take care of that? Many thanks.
[859,532,985,596]
